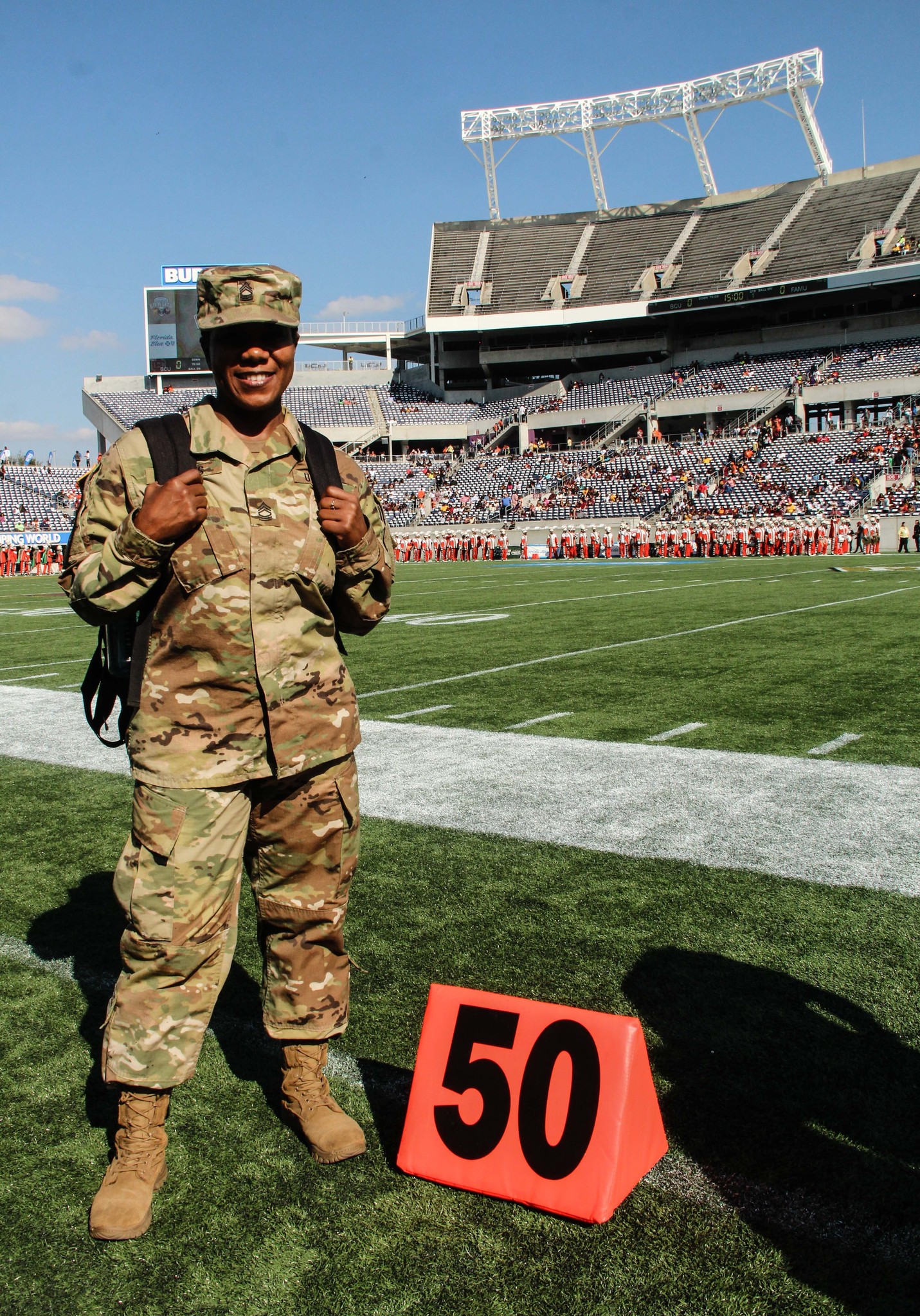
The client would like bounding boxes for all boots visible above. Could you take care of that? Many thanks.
[88,1087,169,1239]
[275,1040,366,1164]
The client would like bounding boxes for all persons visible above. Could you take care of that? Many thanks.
[63,264,396,1240]
[0,234,920,578]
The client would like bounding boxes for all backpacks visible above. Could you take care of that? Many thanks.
[79,414,345,746]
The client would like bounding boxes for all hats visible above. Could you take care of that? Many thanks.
[196,264,303,329]
